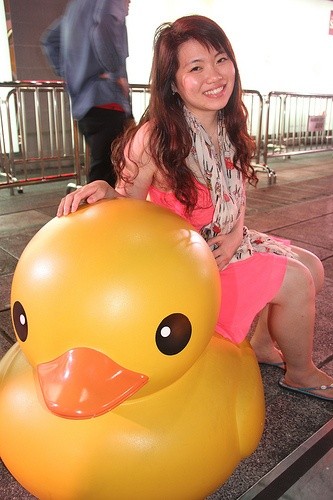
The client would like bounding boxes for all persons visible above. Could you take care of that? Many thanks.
[57,14,333,403]
[39,0,134,205]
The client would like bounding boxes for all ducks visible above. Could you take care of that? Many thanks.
[0,198,266,500]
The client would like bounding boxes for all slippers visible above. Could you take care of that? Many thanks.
[279,377,333,400]
[257,353,286,369]
